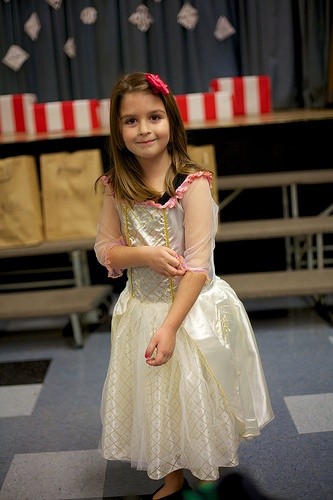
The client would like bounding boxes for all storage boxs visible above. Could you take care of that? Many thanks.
[187,145,218,205]
[41,149,105,242]
[34,99,100,132]
[209,75,271,115]
[174,92,232,124]
[0,93,37,135]
[0,156,42,249]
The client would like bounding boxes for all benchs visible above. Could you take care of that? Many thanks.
[219,268,333,326]
[0,285,113,348]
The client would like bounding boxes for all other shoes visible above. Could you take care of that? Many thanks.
[143,476,192,500]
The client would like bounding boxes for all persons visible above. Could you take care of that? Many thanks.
[91,71,275,500]
